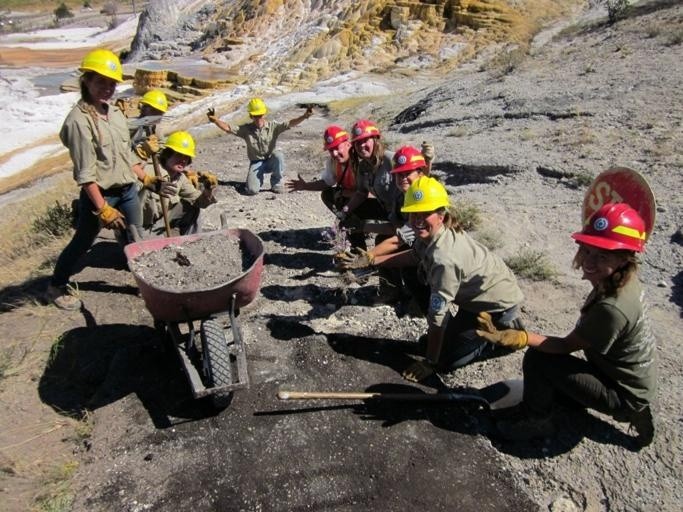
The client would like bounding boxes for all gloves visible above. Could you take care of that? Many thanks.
[402,355,436,381]
[421,140,434,161]
[90,199,127,230]
[206,107,218,123]
[134,135,160,161]
[474,309,528,350]
[341,246,376,272]
[199,172,218,190]
[303,104,315,120]
[143,173,179,199]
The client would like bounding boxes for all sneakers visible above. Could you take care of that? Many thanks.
[44,282,84,312]
[510,315,527,331]
[270,184,287,193]
[498,414,556,443]
[628,402,657,447]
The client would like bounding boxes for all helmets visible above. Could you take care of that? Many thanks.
[246,98,268,117]
[399,176,450,214]
[78,48,124,85]
[138,89,169,114]
[389,143,428,175]
[322,126,350,151]
[572,201,648,255]
[351,118,382,144]
[163,131,196,160]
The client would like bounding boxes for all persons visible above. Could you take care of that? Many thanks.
[207,98,314,195]
[333,177,525,384]
[341,121,434,254]
[46,49,178,311]
[284,126,358,221]
[112,131,218,258]
[131,90,168,160]
[475,202,656,448]
[338,146,429,256]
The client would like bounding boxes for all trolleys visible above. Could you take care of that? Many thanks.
[121,213,268,407]
